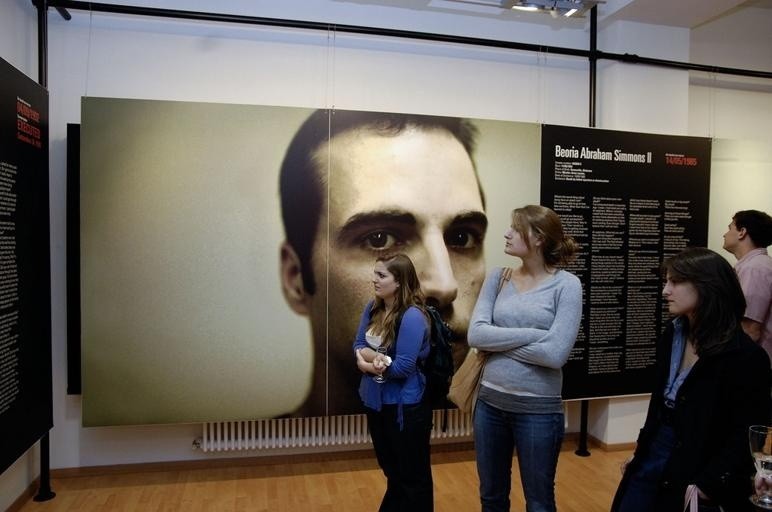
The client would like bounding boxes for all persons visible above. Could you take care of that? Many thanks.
[611,248,755,512]
[276,110,489,421]
[723,210,772,361]
[353,254,434,512]
[467,204,583,511]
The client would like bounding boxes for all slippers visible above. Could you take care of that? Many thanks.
[369,303,458,403]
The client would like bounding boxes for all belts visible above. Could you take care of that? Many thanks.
[748,424,772,509]
[372,347,390,384]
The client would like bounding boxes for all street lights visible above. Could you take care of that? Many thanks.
[446,347,490,421]
